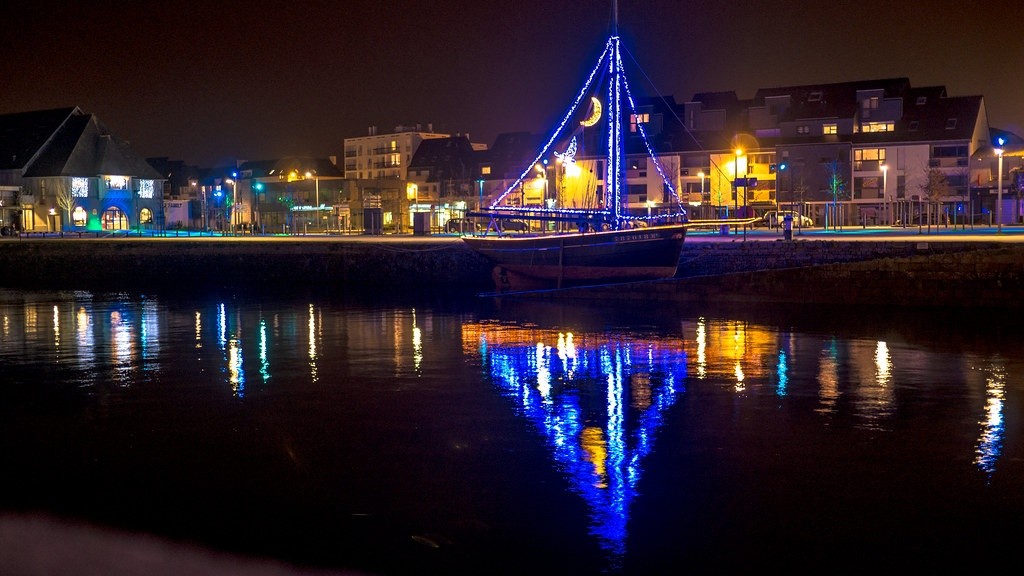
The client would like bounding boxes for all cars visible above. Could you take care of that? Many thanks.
[488,216,528,232]
[894,214,951,226]
[445,218,481,234]
[590,220,647,230]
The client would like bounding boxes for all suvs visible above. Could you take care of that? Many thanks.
[763,210,813,229]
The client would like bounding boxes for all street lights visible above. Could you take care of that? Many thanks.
[542,158,548,231]
[770,163,787,232]
[193,178,208,233]
[697,172,704,218]
[251,184,263,234]
[881,166,889,226]
[411,184,418,213]
[226,180,239,237]
[994,149,1005,234]
[306,172,319,229]
[734,147,742,237]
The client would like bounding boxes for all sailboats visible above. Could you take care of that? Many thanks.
[437,2,771,282]
